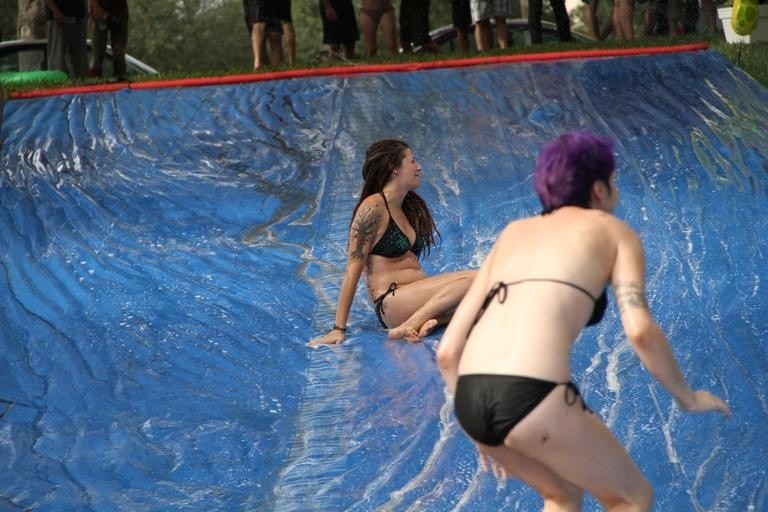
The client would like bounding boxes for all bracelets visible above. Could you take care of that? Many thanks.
[333,325,347,331]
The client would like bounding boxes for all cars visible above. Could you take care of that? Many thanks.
[398,18,594,53]
[0,39,158,76]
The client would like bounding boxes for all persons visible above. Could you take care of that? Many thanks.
[528,0,580,45]
[432,135,732,512]
[243,1,295,72]
[45,1,134,82]
[318,1,444,61]
[451,1,522,57]
[303,141,477,348]
[583,1,726,42]
